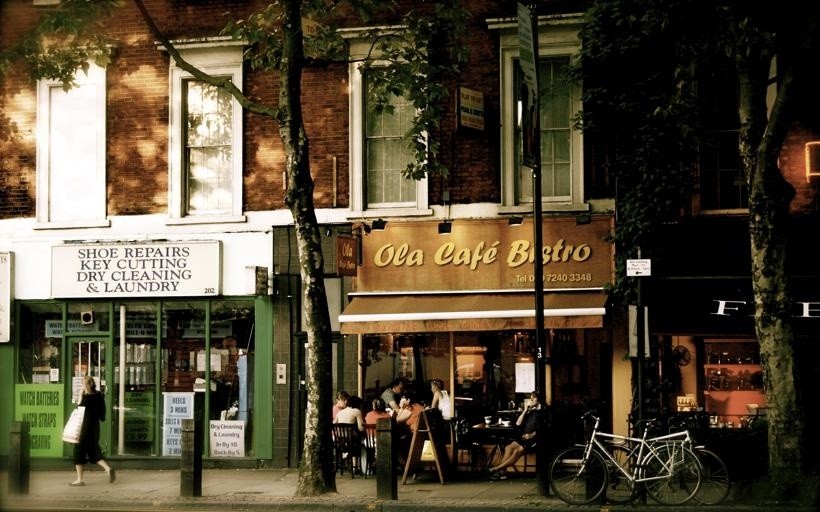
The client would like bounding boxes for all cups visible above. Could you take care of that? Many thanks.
[484,416,492,424]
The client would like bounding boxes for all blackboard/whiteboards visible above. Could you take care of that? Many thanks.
[423,407,455,483]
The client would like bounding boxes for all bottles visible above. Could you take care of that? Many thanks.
[728,421,734,429]
[738,418,747,428]
[709,416,726,428]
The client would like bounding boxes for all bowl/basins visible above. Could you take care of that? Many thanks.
[502,420,510,426]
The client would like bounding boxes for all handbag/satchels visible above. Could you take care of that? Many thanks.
[62,406,85,443]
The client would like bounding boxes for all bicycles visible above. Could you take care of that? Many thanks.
[549,408,731,506]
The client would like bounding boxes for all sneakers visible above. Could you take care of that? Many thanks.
[488,467,507,480]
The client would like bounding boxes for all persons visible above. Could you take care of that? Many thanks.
[332,377,452,476]
[488,390,549,478]
[68,376,115,486]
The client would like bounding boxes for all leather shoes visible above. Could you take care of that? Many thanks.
[109,468,115,483]
[69,482,84,486]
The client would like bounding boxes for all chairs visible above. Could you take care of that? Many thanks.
[330,423,377,481]
[448,416,487,477]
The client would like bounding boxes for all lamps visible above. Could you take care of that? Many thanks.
[508,214,524,226]
[576,210,592,226]
[372,218,387,232]
[437,218,454,235]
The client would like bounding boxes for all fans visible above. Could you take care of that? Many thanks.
[673,345,692,367]
